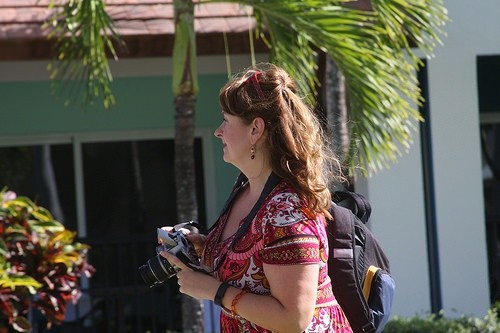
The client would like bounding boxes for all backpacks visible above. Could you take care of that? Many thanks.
[325,189,395,333]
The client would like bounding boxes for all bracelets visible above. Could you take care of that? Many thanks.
[231,289,252,316]
[213,282,233,307]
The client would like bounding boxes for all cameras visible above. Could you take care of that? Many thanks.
[138,226,200,287]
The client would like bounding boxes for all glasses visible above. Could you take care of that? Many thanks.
[243,70,266,101]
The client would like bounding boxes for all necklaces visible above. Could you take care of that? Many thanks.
[234,145,270,191]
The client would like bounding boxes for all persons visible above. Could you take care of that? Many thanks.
[159,62,355,333]
